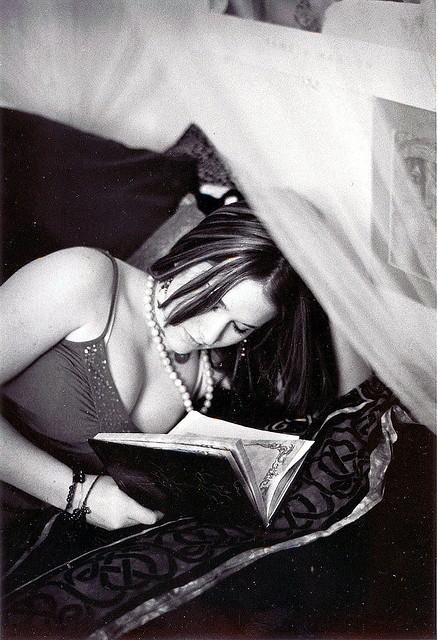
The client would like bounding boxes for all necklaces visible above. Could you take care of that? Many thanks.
[141,274,214,414]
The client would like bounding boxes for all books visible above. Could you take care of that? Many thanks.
[86,409,317,530]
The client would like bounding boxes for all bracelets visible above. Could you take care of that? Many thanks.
[59,466,104,527]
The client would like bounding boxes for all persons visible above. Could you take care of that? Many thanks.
[0,200,325,533]
